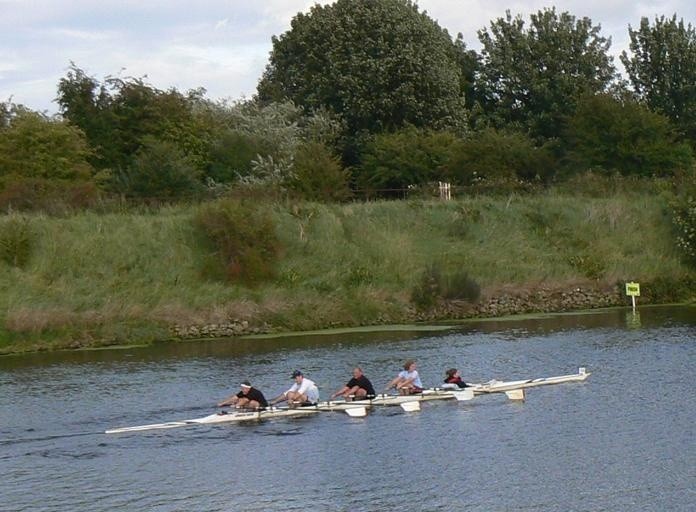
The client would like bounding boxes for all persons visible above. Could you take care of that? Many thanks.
[265,369,319,409]
[384,359,423,395]
[443,368,475,388]
[216,379,268,412]
[328,367,376,401]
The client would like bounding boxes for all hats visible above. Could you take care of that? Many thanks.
[240,382,251,389]
[290,369,303,379]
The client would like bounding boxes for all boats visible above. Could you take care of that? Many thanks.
[104,369,593,435]
